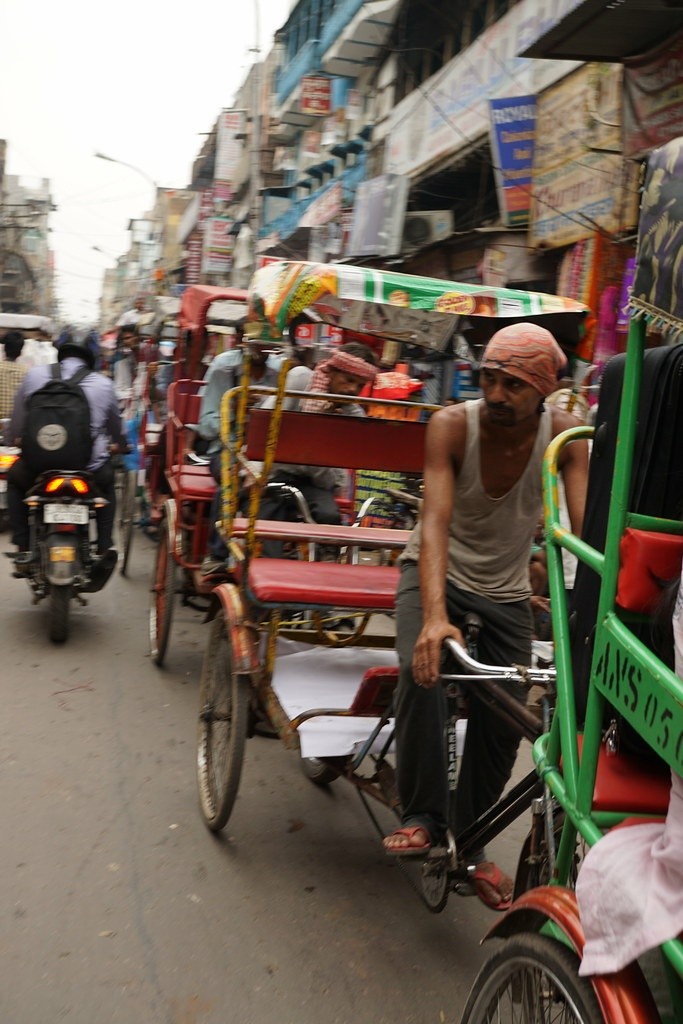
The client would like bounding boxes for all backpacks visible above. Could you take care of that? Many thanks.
[21,363,95,473]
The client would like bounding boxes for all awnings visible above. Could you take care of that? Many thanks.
[513,0,682,62]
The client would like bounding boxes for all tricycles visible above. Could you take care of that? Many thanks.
[460,136,682,1017]
[197,256,591,915]
[97,287,387,666]
[0,313,55,535]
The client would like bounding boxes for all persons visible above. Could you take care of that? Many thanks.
[0,295,318,576]
[396,323,590,910]
[6,324,132,556]
[242,342,380,630]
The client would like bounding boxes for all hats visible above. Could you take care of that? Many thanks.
[235,322,286,348]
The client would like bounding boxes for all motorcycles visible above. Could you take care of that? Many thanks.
[14,469,109,646]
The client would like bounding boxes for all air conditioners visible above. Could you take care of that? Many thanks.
[403,209,454,252]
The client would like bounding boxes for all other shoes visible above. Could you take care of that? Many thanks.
[202,554,227,575]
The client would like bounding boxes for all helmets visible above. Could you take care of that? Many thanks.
[57,325,99,364]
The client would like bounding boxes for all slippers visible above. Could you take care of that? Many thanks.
[467,858,515,910]
[382,820,444,856]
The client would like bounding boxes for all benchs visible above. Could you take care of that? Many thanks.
[140,360,683,829]
